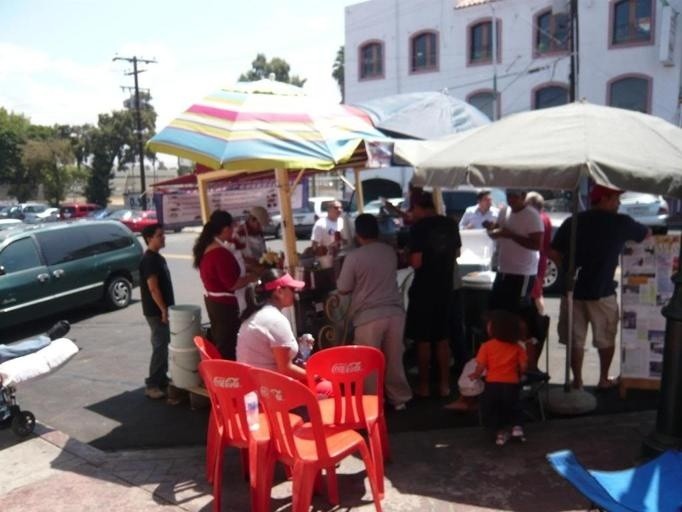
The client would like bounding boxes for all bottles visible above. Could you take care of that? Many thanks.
[244,390,260,430]
[335,230,341,243]
[297,333,313,363]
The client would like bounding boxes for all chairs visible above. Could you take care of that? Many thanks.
[191,335,393,511]
[468,314,554,427]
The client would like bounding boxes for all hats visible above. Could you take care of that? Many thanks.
[588,183,627,203]
[254,270,306,294]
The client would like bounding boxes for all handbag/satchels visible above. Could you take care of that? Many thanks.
[491,251,501,272]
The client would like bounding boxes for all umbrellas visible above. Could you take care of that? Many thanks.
[351,86,501,145]
[142,76,387,278]
[406,96,681,415]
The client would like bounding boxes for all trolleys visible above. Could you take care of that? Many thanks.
[0,376,38,442]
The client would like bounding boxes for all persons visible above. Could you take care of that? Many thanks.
[554,189,654,394]
[137,221,182,411]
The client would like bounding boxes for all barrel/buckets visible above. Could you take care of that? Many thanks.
[166,303,203,350]
[166,343,203,390]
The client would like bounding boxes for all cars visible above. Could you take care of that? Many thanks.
[0,197,159,329]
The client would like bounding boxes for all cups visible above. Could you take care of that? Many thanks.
[259,250,284,269]
[299,255,334,269]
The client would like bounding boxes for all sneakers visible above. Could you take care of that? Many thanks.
[393,402,407,411]
[510,425,525,438]
[143,378,175,399]
[571,376,620,389]
[495,430,509,447]
